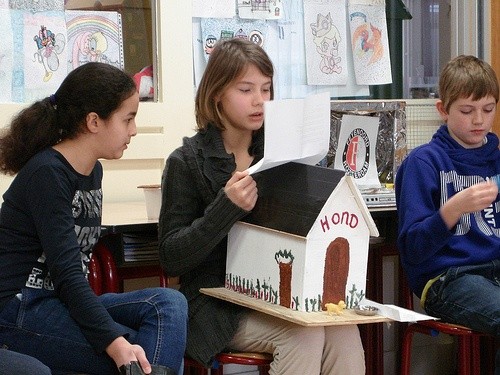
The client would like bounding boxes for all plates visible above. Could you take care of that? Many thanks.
[354,305,379,316]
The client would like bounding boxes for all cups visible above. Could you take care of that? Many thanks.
[143,188,161,220]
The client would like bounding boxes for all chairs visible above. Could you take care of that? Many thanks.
[159,273,274,375]
[380,247,497,375]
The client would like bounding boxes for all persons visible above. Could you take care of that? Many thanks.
[159,39,367,375]
[0,62,188,375]
[395,54,500,375]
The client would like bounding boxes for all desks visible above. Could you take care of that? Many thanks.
[85,210,397,375]
